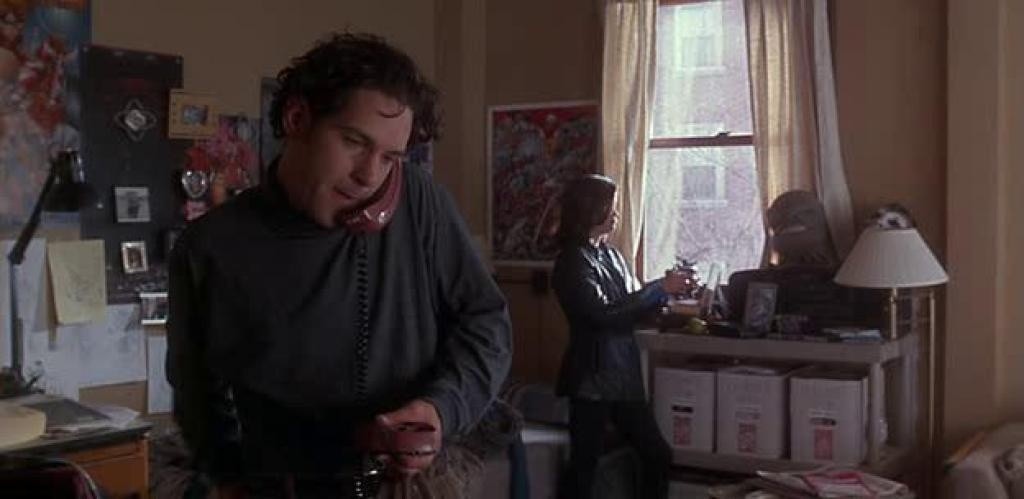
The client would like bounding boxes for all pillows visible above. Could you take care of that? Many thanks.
[505,383,572,424]
[991,436,1024,497]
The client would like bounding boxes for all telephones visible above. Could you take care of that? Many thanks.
[341,157,402,234]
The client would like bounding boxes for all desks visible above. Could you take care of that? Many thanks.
[1,392,152,498]
[631,325,919,498]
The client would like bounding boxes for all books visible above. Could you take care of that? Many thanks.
[802,470,875,499]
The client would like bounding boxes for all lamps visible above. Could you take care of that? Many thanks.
[4,151,97,397]
[832,227,949,341]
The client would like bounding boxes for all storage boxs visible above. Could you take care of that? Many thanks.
[720,364,788,461]
[653,365,715,462]
[788,370,893,473]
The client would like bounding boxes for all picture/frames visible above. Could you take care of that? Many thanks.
[168,88,224,139]
[139,291,171,325]
[113,96,158,143]
[114,185,153,222]
[740,281,779,330]
[122,240,149,273]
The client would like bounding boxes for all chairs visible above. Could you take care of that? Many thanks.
[942,413,1024,496]
[0,454,101,498]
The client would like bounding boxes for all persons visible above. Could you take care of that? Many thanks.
[164,29,515,498]
[549,174,701,498]
